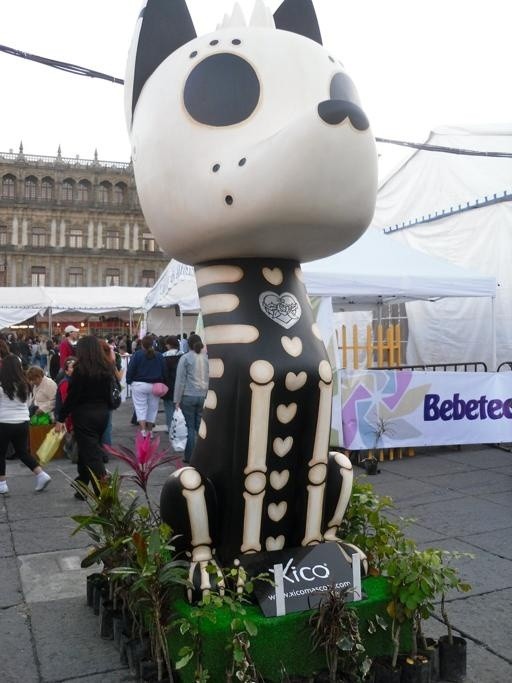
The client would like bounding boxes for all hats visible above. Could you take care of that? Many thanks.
[64,326,80,333]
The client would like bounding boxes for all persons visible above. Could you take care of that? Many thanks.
[0,324,209,502]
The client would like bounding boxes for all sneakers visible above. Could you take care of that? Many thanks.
[150,431,154,439]
[0,480,9,494]
[140,429,147,438]
[35,471,52,491]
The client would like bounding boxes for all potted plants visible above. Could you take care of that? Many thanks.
[316,512,480,681]
[58,427,195,678]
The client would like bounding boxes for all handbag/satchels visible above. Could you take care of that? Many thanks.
[110,376,121,409]
[152,383,169,398]
[36,426,64,466]
[169,407,188,452]
[30,413,52,426]
[29,403,38,417]
